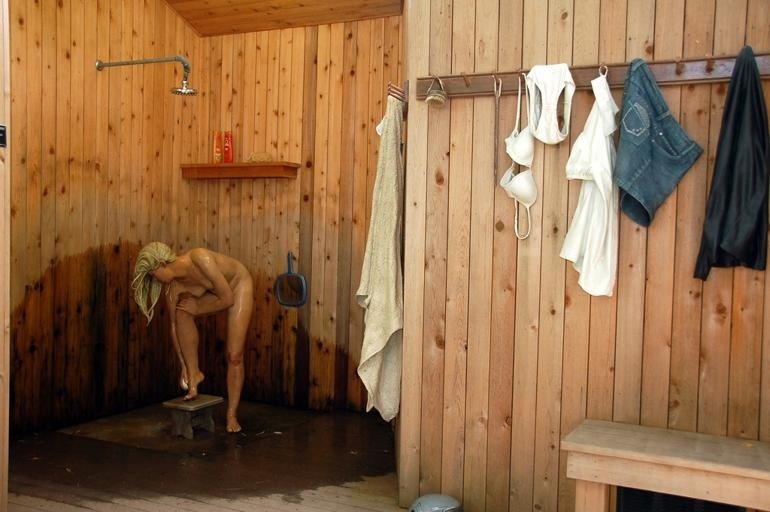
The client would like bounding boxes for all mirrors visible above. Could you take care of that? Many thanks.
[273,251,307,308]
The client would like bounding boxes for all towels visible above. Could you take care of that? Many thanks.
[353,83,409,426]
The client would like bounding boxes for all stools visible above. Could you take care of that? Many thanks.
[159,392,224,438]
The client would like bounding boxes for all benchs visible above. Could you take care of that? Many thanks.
[558,415,770,512]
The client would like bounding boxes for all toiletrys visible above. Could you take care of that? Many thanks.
[224,131,233,162]
[212,131,222,164]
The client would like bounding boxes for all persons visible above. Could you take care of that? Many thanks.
[131,241,253,432]
